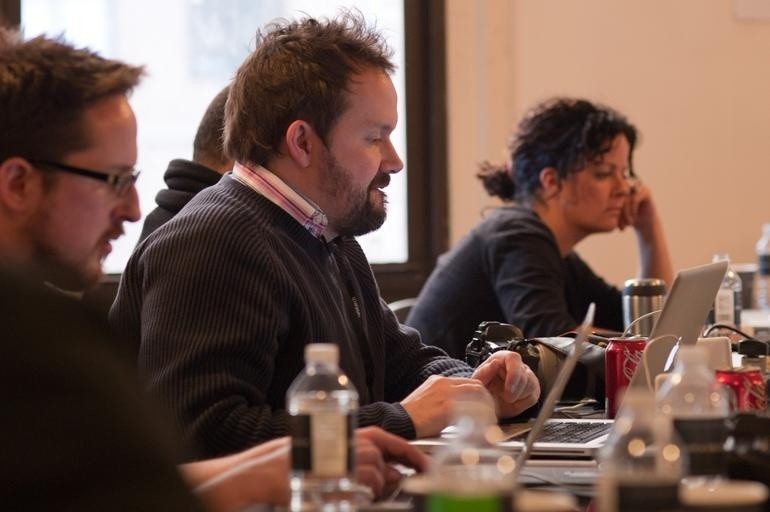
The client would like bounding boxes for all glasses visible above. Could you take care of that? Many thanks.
[41,167,141,196]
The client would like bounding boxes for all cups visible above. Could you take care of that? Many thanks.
[399,465,515,512]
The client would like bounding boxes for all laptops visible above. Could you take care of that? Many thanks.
[272,302,595,512]
[406,260,728,457]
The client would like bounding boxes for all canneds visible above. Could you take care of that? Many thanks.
[605,335,648,420]
[715,366,767,414]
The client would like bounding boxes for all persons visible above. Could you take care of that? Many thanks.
[133,79,237,246]
[0,23,435,512]
[407,90,676,360]
[109,8,541,437]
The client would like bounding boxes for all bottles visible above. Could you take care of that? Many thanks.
[287,343,358,512]
[756,224,770,309]
[706,254,743,333]
[622,278,666,337]
[607,344,732,512]
